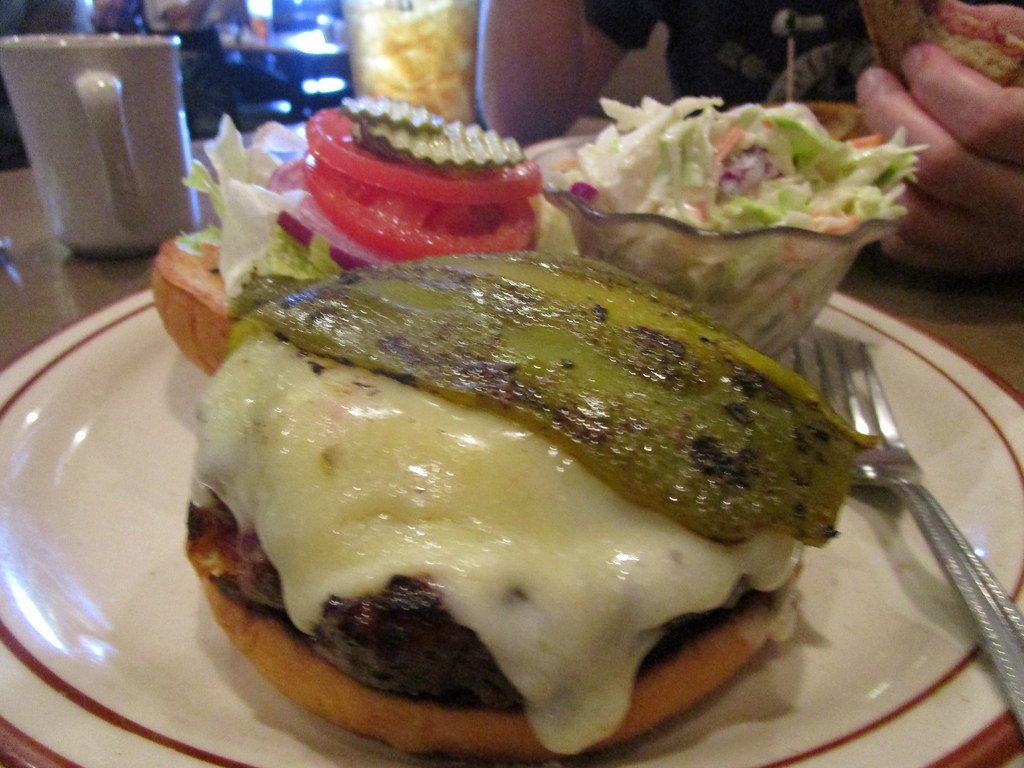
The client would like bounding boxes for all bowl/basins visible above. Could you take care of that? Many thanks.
[539,167,904,367]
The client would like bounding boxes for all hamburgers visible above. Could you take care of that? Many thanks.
[155,98,877,758]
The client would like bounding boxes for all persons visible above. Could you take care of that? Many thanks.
[475,0,1024,287]
[1,0,253,168]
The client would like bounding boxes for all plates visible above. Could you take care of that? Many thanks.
[0,280,1024,768]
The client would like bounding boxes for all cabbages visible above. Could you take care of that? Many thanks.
[571,91,929,352]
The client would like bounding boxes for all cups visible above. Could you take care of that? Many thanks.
[0,33,203,262]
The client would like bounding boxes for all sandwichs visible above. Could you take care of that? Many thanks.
[860,0,1024,91]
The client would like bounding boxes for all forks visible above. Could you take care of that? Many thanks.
[788,337,1024,768]
